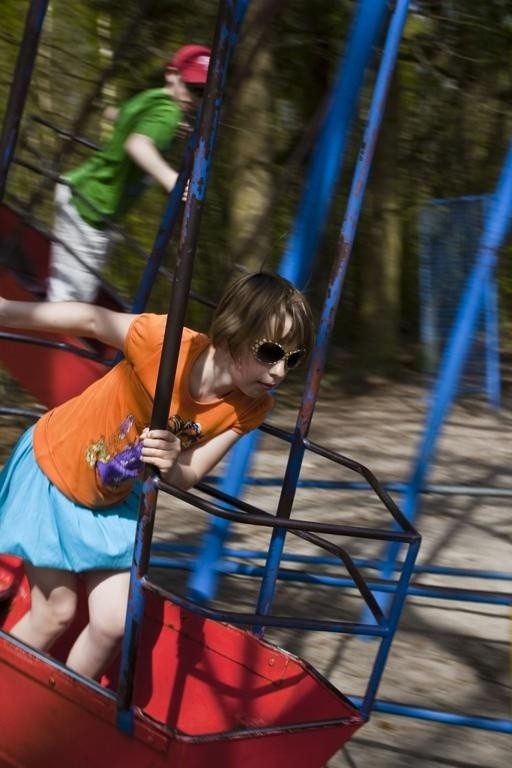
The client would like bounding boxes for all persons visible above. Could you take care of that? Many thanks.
[0,265,319,681]
[46,43,212,303]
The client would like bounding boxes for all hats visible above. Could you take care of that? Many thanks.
[165,43,212,84]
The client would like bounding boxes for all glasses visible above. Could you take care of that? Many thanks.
[251,338,306,371]
